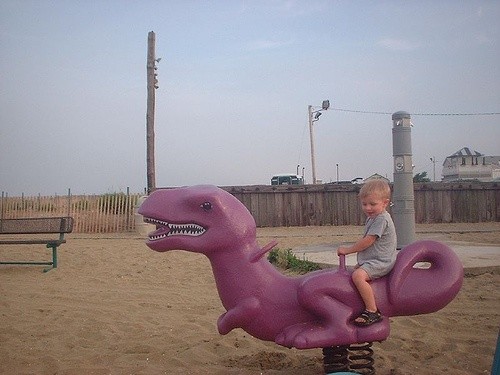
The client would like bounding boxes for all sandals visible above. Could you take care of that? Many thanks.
[353,307,384,327]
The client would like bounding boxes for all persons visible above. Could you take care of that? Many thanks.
[337,180,397,326]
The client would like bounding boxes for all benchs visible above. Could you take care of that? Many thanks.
[0,217,73,268]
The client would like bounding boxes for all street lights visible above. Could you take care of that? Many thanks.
[302,167,305,180]
[336,164,338,184]
[297,165,300,175]
